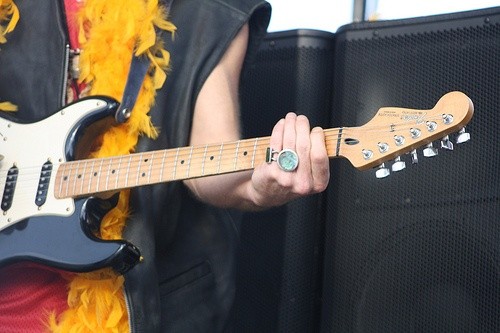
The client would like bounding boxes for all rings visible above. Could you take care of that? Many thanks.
[277,150,299,172]
[266,148,277,163]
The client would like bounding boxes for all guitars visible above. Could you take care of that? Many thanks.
[0,89,476,277]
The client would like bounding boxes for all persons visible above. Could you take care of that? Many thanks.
[1,1,330,332]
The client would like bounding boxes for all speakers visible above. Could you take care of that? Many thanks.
[318,5,500,332]
[148,27,336,333]
